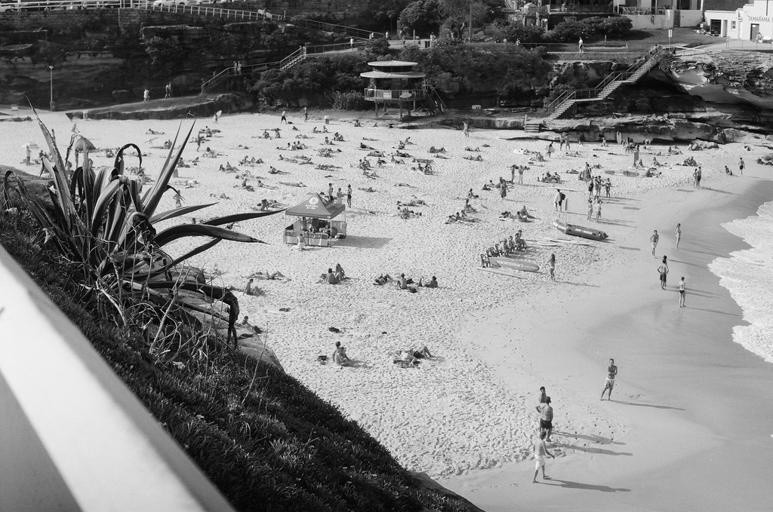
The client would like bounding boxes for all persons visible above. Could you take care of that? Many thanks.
[516,37,521,46]
[599,358,617,402]
[236,61,243,75]
[532,430,555,483]
[143,88,151,103]
[11,105,697,297]
[697,166,702,185]
[537,387,549,403]
[678,276,687,308]
[628,43,663,71]
[737,157,746,175]
[331,341,445,369]
[241,315,263,335]
[612,4,673,24]
[535,396,553,443]
[348,27,438,49]
[164,82,171,99]
[724,165,732,175]
[578,36,585,53]
[232,61,237,74]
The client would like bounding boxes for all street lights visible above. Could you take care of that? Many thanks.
[668,26,672,53]
[48,65,56,112]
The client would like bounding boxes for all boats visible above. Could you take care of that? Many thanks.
[553,218,608,241]
